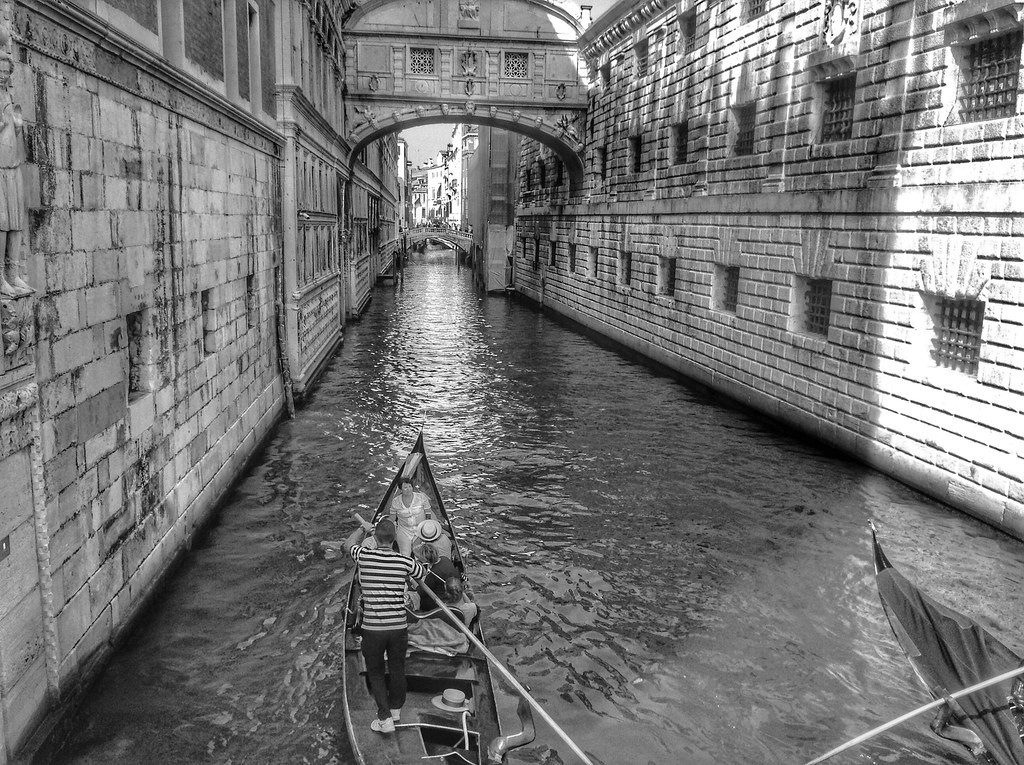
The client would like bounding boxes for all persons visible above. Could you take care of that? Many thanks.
[389,478,431,556]
[419,544,458,610]
[418,222,473,233]
[417,520,452,561]
[344,519,426,733]
[442,577,481,632]
[0,52,38,296]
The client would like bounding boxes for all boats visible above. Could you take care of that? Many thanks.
[866,518,1024,765]
[341,424,538,765]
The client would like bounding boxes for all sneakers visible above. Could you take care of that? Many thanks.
[390,709,400,721]
[371,717,395,733]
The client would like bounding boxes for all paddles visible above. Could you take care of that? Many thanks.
[354,510,595,765]
[804,666,1024,765]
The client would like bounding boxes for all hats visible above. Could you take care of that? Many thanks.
[417,519,441,541]
[431,688,470,712]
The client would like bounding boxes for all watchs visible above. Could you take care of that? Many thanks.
[360,525,365,533]
